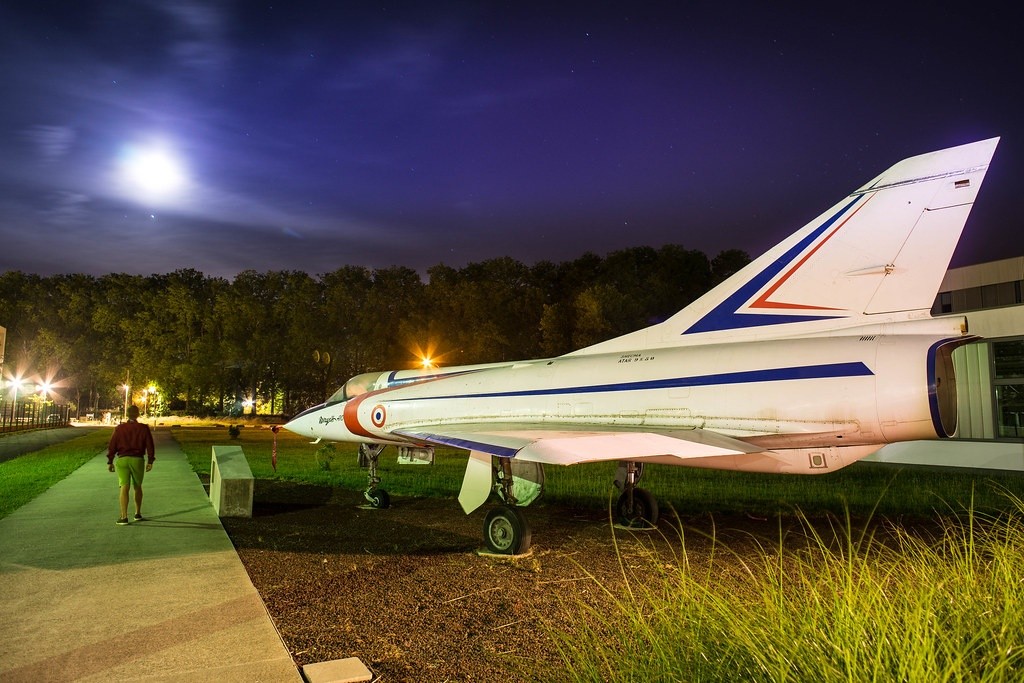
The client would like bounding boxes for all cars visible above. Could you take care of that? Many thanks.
[46,414,62,421]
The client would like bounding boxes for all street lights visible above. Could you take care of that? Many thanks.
[142,388,147,417]
[42,382,50,403]
[123,383,128,420]
[12,377,20,422]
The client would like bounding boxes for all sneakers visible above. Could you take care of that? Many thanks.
[134,514,142,521]
[116,517,129,525]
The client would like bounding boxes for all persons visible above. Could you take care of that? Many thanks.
[106,405,156,525]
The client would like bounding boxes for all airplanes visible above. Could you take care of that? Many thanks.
[271,135,1003,557]
